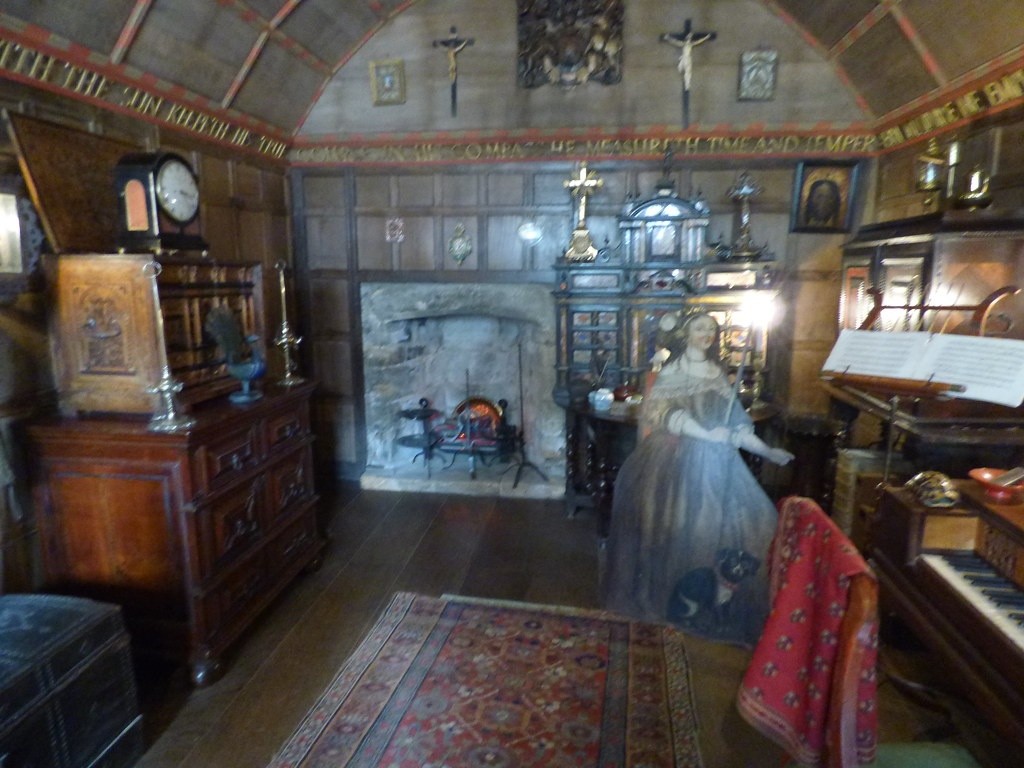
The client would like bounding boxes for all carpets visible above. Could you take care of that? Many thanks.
[266,589,706,768]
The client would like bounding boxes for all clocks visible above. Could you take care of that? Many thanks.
[115,148,212,258]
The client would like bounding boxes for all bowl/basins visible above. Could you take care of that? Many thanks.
[969,466,1024,498]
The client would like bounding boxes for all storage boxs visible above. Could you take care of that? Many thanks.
[0,107,272,421]
[0,594,145,768]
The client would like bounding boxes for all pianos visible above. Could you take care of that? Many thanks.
[869,462,1024,768]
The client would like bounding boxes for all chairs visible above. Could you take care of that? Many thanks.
[737,495,983,768]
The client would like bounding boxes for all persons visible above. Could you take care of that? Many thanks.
[437,39,468,84]
[601,313,794,645]
[664,33,711,89]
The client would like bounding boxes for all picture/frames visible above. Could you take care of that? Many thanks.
[788,156,865,233]
[368,58,406,106]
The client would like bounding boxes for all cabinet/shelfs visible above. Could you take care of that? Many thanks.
[9,377,329,686]
